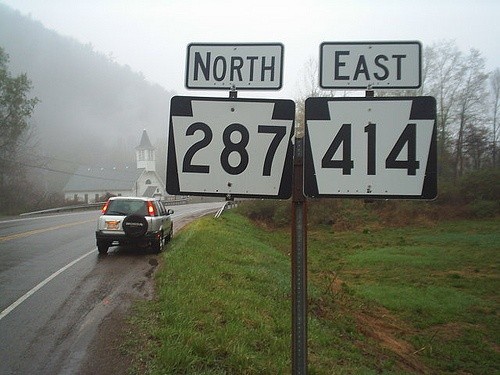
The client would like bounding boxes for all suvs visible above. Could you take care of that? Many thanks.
[96,196,176,253]
